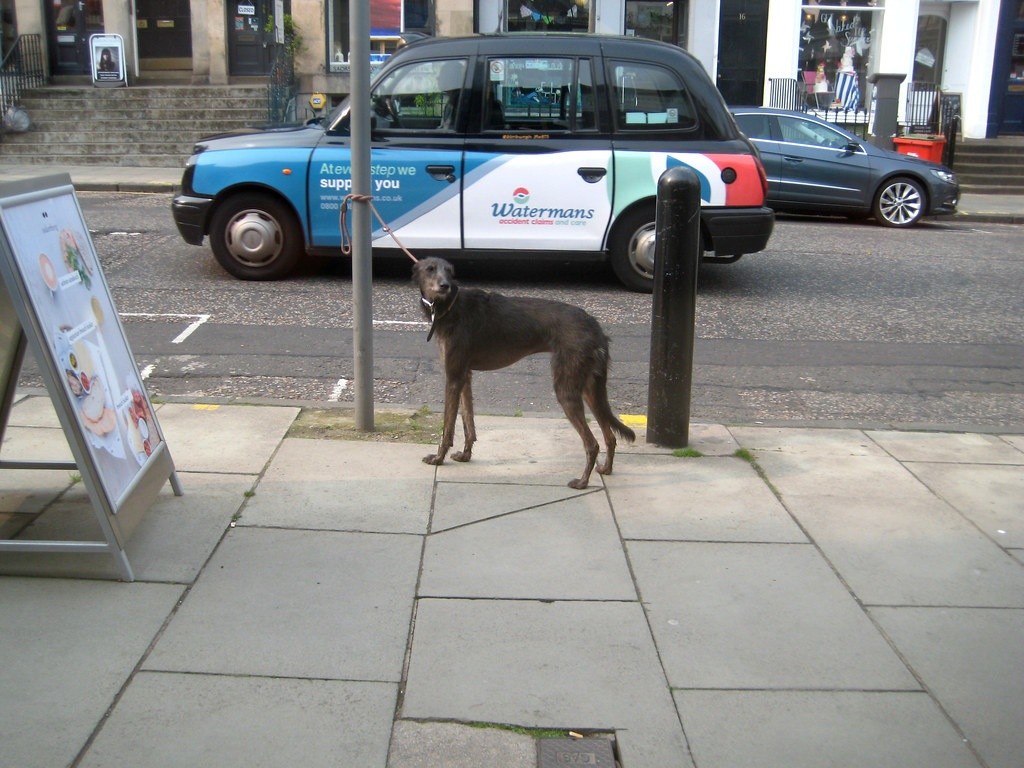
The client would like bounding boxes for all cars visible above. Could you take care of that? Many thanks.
[727,105,961,229]
[170,34,774,294]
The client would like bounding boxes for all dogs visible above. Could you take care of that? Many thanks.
[410,256,636,490]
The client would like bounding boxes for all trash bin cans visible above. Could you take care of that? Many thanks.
[891,132,949,164]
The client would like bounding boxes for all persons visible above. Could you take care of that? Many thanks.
[100,48,115,72]
[432,62,463,130]
